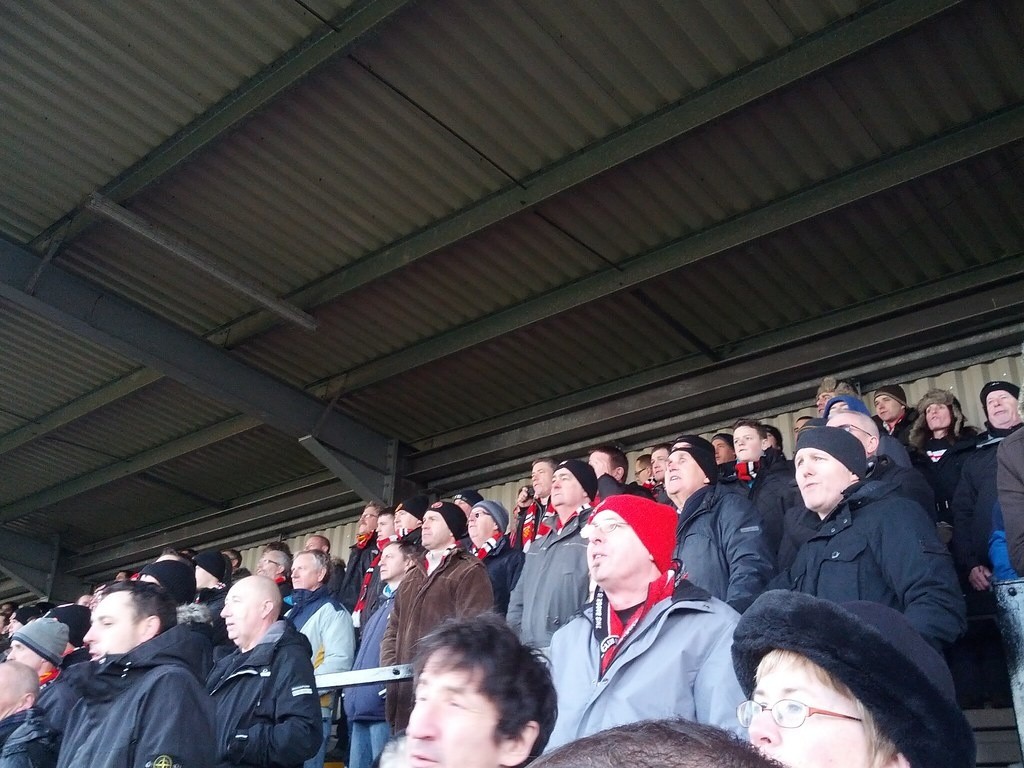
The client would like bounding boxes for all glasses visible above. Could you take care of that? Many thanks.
[838,425,874,437]
[361,514,377,517]
[588,520,630,533]
[260,559,279,565]
[635,468,646,476]
[736,699,862,728]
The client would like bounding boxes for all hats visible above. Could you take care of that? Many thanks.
[470,500,510,533]
[796,426,867,480]
[394,496,429,521]
[137,560,195,605]
[588,494,677,574]
[711,433,734,448]
[454,490,484,507]
[764,424,782,447]
[731,589,978,768]
[425,501,467,541]
[816,376,862,401]
[553,460,598,501]
[980,381,1020,400]
[874,384,907,406]
[908,389,964,449]
[192,550,225,581]
[10,601,92,667]
[669,448,719,484]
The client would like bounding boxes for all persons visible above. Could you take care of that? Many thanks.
[0,377,1024,768]
